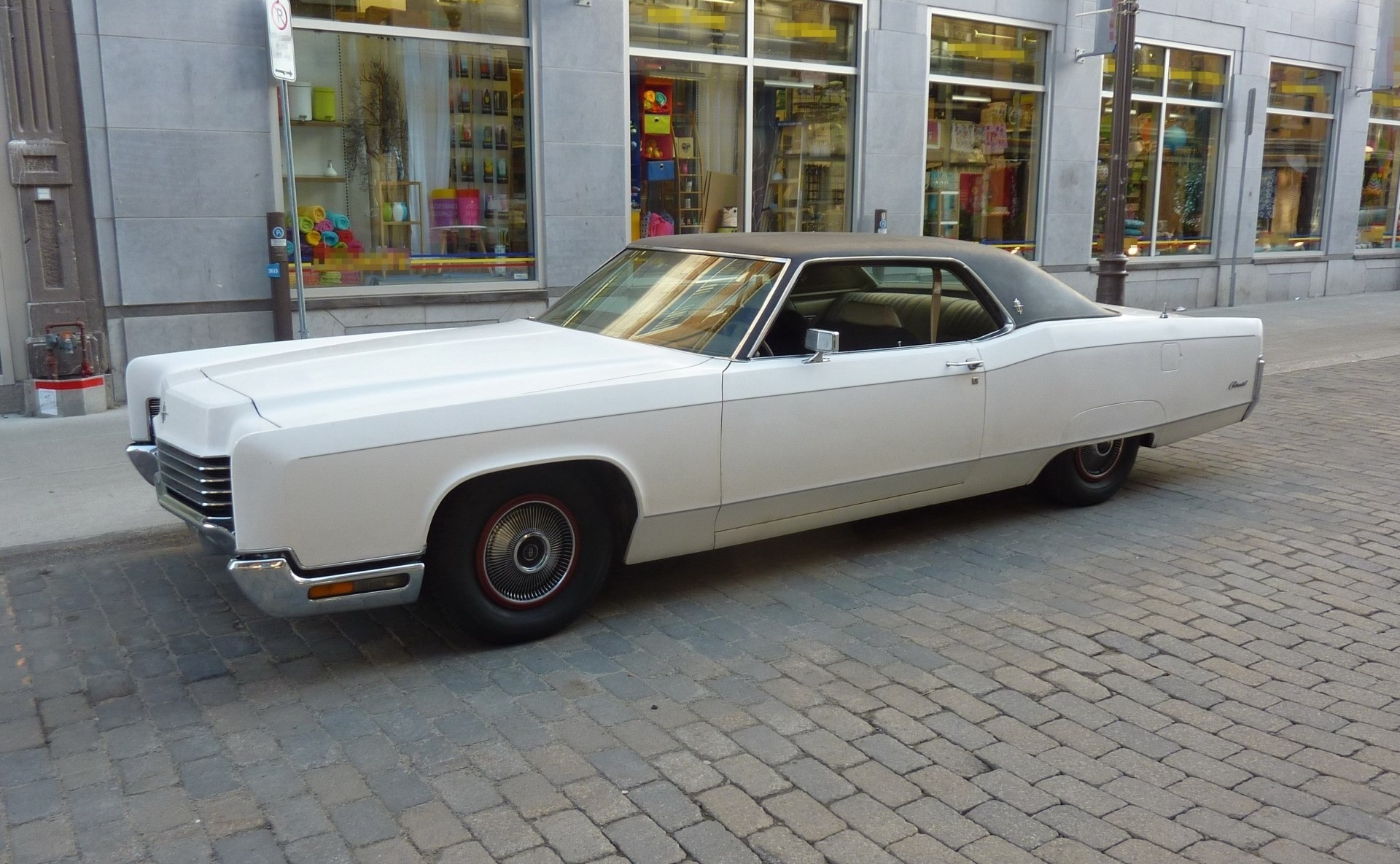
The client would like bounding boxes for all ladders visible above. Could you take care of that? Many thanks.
[757,123,808,233]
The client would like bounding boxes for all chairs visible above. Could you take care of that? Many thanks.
[725,307,807,355]
[826,319,917,350]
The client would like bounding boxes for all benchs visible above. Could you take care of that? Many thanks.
[819,291,996,342]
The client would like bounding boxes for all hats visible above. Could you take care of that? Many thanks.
[1286,153,1309,174]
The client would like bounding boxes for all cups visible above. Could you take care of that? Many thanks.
[685,181,692,192]
[685,199,691,209]
[682,164,687,174]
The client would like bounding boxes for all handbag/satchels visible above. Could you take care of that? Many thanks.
[1362,158,1383,196]
[1357,213,1386,242]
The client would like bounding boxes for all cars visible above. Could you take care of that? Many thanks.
[126,225,1268,647]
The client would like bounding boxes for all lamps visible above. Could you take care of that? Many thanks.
[647,70,707,83]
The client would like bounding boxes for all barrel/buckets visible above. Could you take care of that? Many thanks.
[432,188,480,226]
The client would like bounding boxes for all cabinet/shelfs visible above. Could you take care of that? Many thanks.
[378,178,426,277]
[927,190,959,236]
[279,119,347,183]
[670,110,705,234]
[777,117,845,231]
[639,75,675,183]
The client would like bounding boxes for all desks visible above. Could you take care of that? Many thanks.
[432,226,494,275]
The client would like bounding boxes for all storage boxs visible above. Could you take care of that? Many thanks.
[644,112,671,135]
[644,160,675,181]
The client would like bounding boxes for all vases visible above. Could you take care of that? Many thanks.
[383,201,408,222]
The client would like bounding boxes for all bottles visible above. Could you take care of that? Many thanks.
[324,162,338,177]
[484,89,490,104]
[461,83,470,101]
[497,60,502,75]
[384,202,408,222]
[500,126,505,143]
